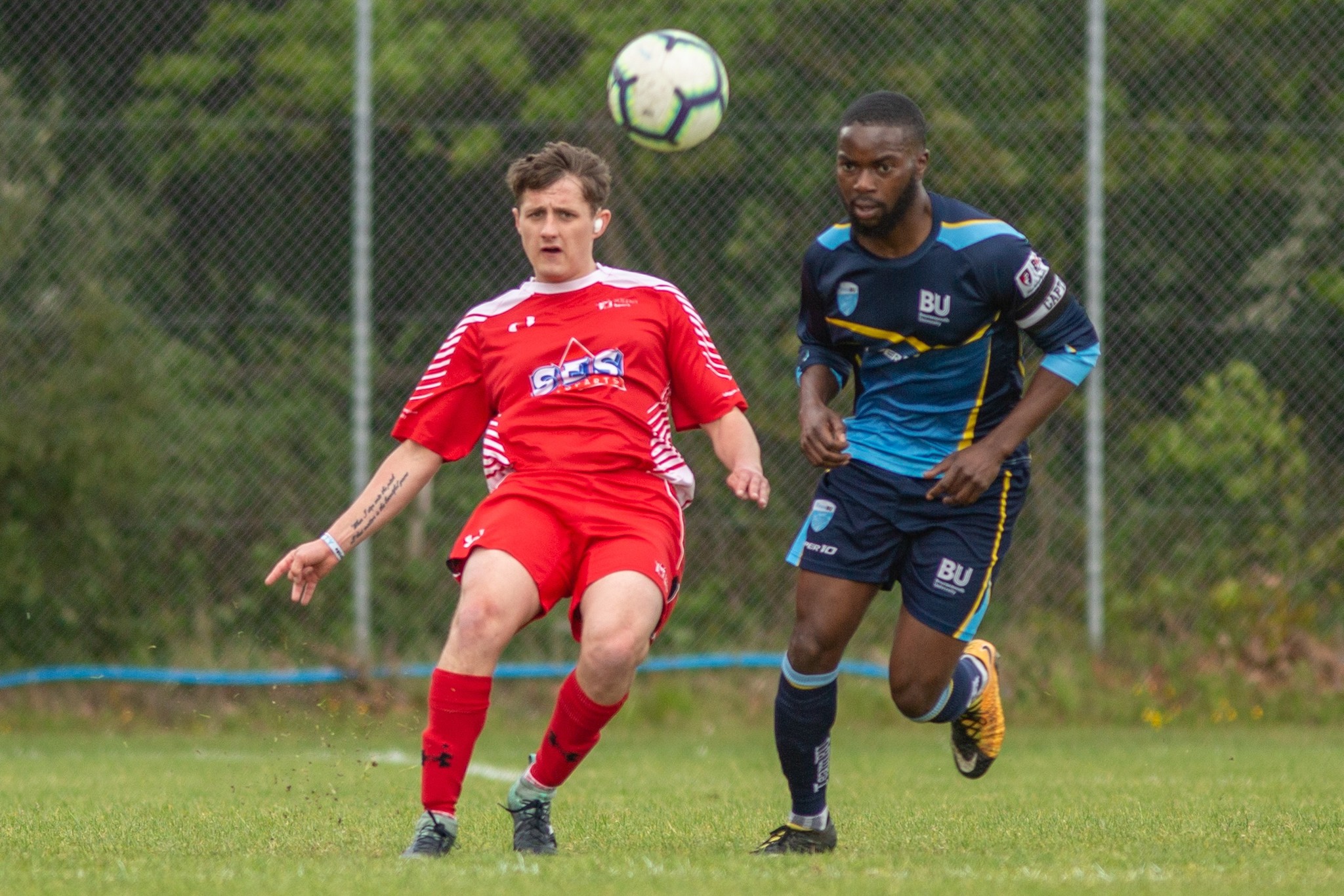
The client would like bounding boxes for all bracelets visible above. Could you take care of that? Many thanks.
[321,532,346,560]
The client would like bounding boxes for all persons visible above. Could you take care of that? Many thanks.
[264,142,771,855]
[751,90,1102,853]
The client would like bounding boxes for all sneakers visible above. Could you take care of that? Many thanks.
[749,812,838,855]
[497,754,558,855]
[402,809,461,860]
[950,639,1005,779]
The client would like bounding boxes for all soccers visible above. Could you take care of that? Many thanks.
[607,29,730,152]
